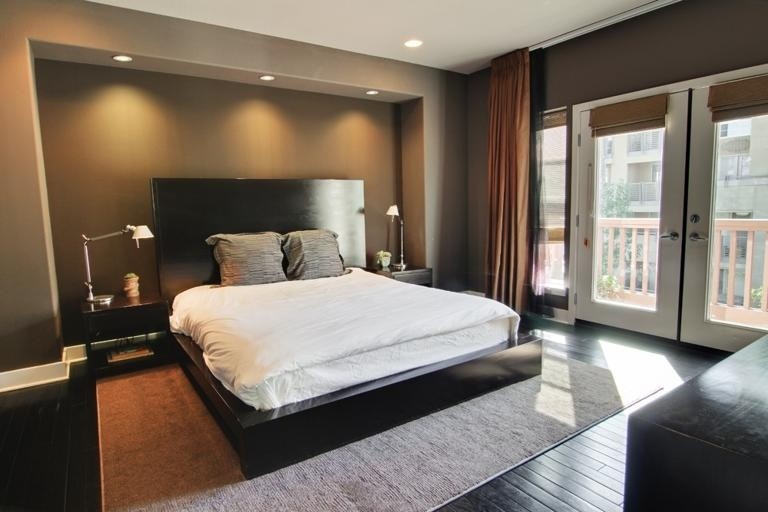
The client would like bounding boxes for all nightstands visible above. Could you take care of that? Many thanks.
[370,263,434,289]
[80,296,173,380]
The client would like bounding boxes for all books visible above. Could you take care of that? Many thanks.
[105,347,154,363]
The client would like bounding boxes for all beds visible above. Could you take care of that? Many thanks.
[151,178,545,477]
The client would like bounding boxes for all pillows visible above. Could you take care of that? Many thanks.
[206,229,353,290]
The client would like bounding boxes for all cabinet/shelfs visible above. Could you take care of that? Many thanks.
[626,337,765,510]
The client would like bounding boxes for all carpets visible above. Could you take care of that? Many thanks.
[95,348,663,510]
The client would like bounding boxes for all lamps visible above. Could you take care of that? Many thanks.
[77,225,157,304]
[386,205,409,269]
